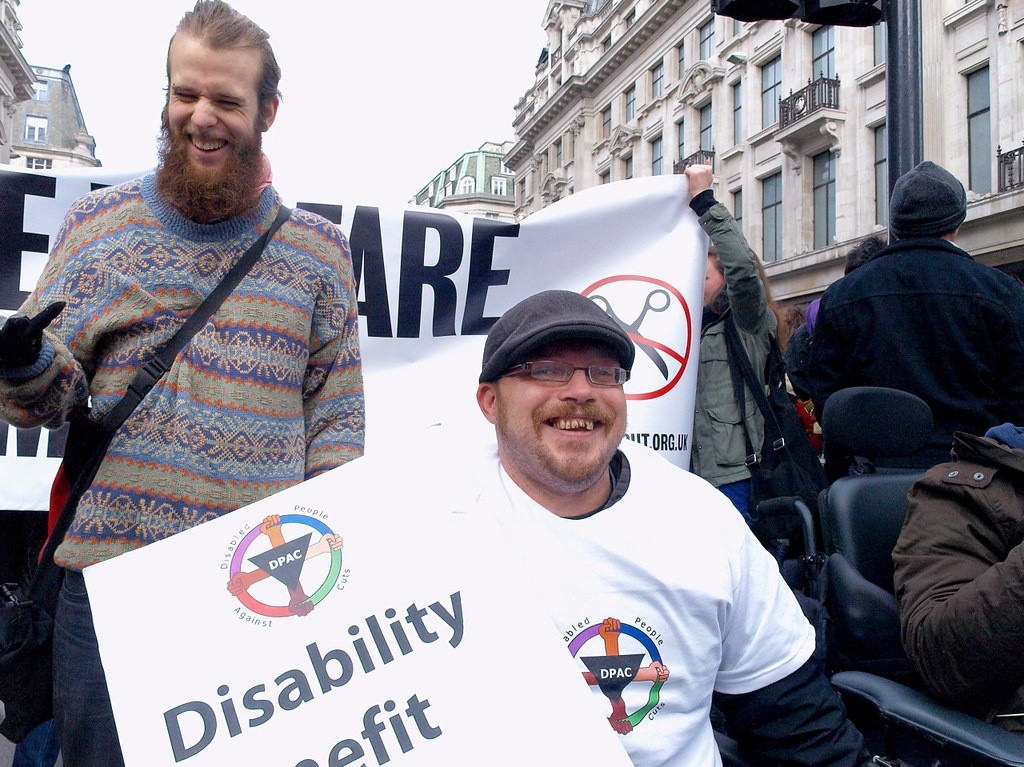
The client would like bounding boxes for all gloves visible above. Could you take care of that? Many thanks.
[0,300,65,366]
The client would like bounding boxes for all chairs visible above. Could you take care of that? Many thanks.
[816,387,946,680]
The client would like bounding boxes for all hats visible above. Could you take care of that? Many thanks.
[889,161,967,236]
[478,290,636,382]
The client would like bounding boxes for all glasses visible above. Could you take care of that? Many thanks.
[503,360,629,388]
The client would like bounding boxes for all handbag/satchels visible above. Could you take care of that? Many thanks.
[758,390,827,555]
[0,530,56,744]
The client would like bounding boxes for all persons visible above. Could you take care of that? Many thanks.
[446,290,876,767]
[682,158,1024,735]
[0,0,364,767]
